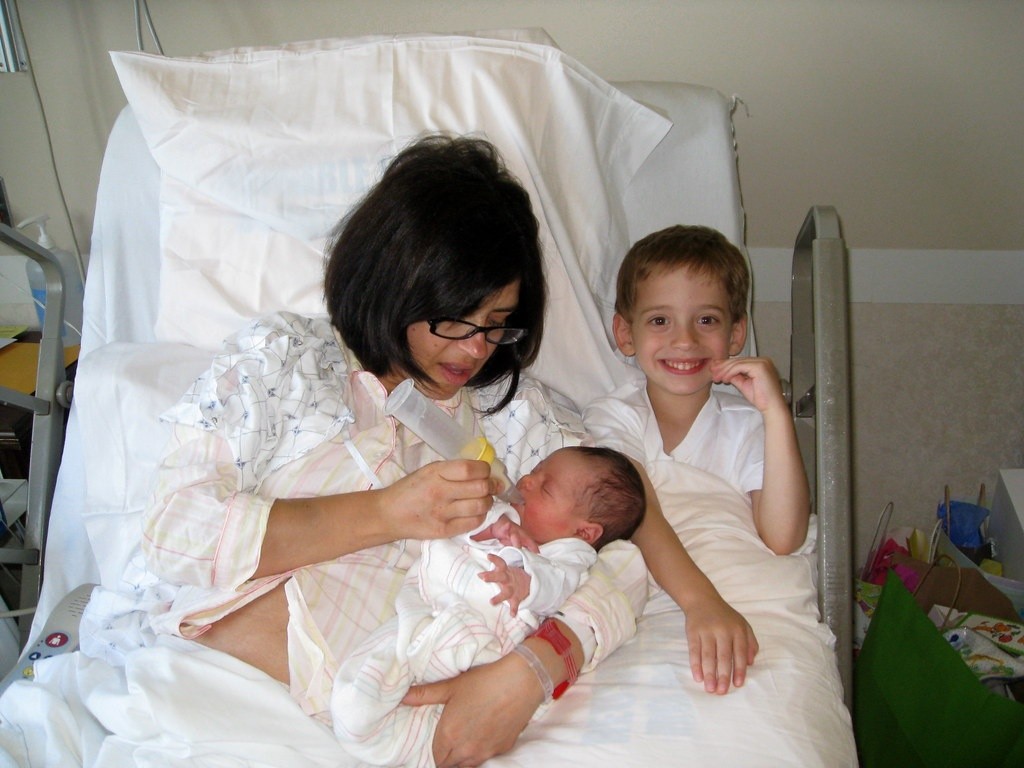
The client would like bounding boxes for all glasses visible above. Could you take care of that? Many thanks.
[426,316,528,346]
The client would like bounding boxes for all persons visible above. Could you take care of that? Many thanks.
[0,130,645,768]
[328,447,647,768]
[585,223,810,696]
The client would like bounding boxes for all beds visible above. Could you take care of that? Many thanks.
[0,81,863,768]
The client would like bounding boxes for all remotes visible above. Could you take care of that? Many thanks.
[0,584,101,698]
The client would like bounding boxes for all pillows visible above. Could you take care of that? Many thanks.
[107,36,674,413]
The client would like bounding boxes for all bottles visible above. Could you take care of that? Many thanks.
[384,375,524,507]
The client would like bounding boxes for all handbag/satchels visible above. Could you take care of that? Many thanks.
[853,484,1024,768]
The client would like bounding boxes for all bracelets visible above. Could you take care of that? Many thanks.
[527,620,577,700]
[513,644,554,706]
